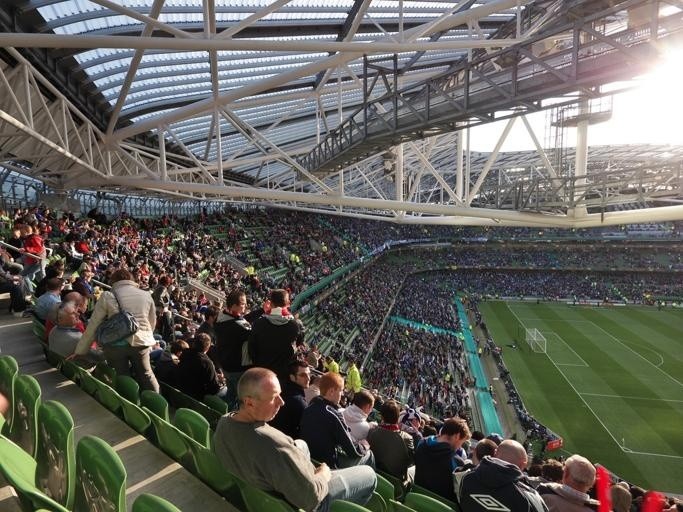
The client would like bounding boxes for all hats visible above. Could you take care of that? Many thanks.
[487,433,504,445]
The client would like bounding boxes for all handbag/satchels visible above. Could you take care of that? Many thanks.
[96,312,139,346]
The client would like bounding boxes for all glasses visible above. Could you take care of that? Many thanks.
[295,373,311,377]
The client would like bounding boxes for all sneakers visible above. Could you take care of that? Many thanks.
[13,312,32,318]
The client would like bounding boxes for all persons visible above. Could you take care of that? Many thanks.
[0,203,570,512]
[570,452,681,511]
[570,221,683,313]
